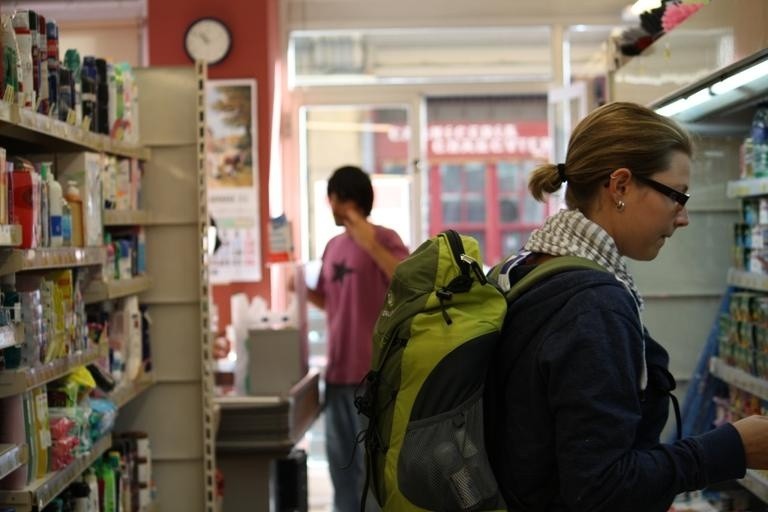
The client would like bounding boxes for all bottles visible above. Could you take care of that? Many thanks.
[47,176,82,251]
[11,7,59,120]
[50,430,153,512]
[59,41,140,145]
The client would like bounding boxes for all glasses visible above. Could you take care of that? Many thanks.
[643,176,691,212]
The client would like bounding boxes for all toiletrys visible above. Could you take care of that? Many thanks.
[74,430,153,512]
[41,160,64,245]
[53,49,142,143]
[120,295,143,368]
[136,228,147,276]
[66,177,84,244]
[105,154,144,213]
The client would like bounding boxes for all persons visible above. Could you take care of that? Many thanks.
[285,163,412,512]
[485,101,768,512]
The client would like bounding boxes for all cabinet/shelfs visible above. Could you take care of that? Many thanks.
[1,93,158,512]
[706,175,767,508]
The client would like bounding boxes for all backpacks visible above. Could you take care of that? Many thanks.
[351,230,608,511]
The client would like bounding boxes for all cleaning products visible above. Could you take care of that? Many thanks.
[8,152,33,248]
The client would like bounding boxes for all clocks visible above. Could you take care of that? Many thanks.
[186,16,230,67]
